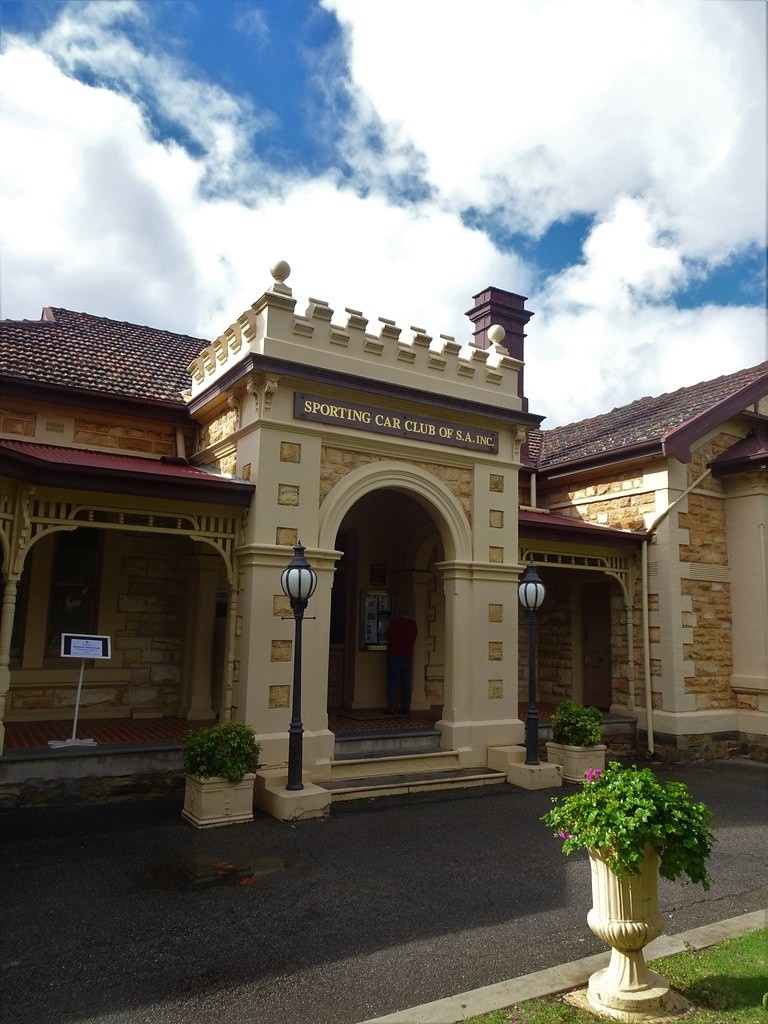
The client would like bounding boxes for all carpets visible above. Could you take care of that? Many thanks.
[339,710,413,721]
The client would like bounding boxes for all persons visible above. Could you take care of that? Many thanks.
[383,601,418,715]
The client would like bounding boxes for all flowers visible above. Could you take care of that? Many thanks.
[540,761,718,893]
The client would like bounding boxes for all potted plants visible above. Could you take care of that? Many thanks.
[546,700,607,785]
[181,720,264,830]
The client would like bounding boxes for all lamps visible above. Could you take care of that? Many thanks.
[518,561,546,610]
[280,539,318,609]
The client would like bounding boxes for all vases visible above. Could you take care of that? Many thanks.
[582,843,673,1011]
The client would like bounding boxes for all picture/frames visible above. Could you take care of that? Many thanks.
[370,564,386,586]
